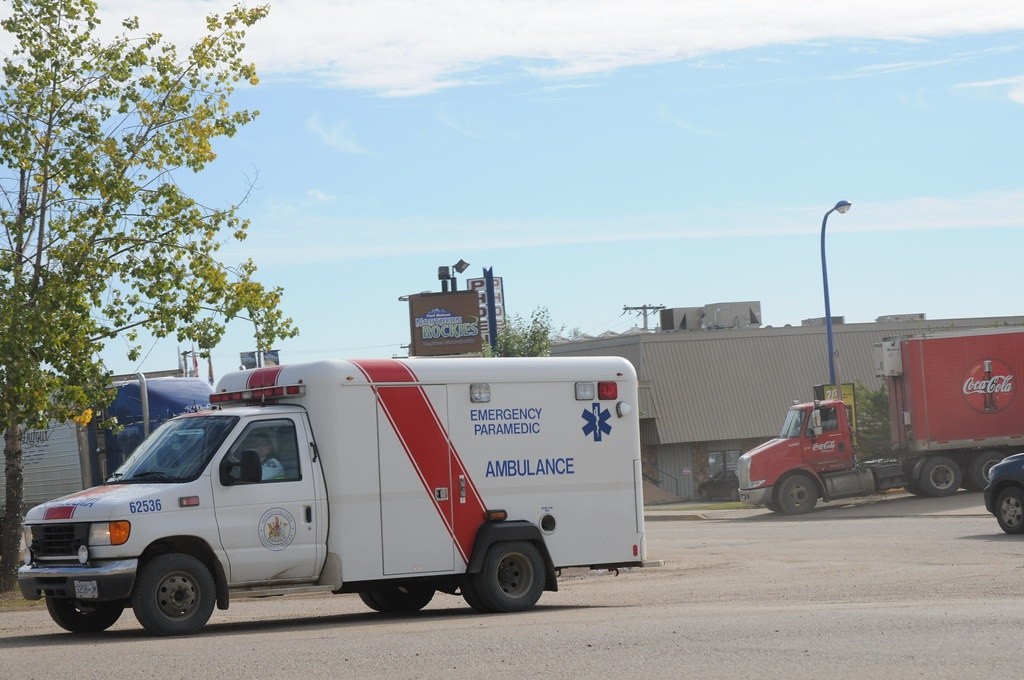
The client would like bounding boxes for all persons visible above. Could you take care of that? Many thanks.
[251,432,285,480]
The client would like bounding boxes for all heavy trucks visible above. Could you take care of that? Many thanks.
[16,357,663,637]
[0,371,214,520]
[737,326,1024,515]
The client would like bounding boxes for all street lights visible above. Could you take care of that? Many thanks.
[820,201,853,384]
[437,259,470,291]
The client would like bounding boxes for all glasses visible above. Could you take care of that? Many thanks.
[252,441,271,447]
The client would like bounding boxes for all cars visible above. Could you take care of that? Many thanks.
[697,470,741,501]
[983,452,1024,536]
